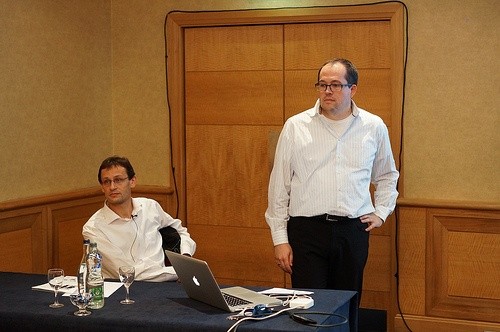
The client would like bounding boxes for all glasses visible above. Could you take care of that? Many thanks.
[101,175,130,188]
[315,83,352,91]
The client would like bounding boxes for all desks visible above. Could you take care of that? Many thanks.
[0,271,359,332]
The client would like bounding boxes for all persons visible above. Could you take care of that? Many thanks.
[82,156,196,282]
[264,57,399,309]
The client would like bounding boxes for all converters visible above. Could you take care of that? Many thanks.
[290,297,315,309]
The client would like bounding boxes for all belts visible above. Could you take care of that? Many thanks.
[292,213,368,223]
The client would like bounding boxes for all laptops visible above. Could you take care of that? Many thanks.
[165,250,284,313]
